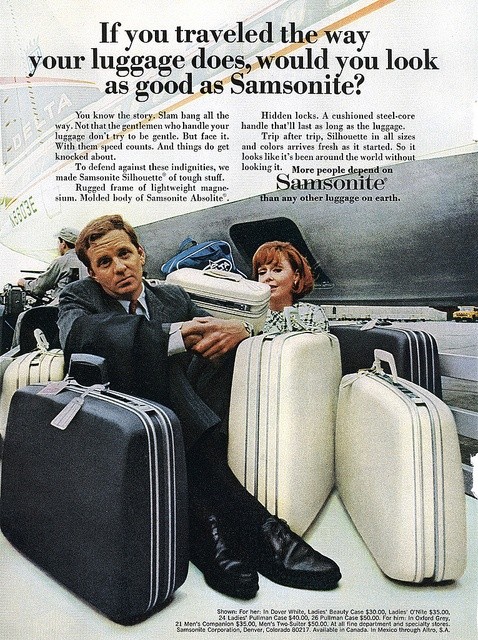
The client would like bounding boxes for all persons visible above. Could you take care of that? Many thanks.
[252,238,331,336]
[9,227,92,358]
[57,215,342,600]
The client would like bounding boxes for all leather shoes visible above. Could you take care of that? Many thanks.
[230,514,342,590]
[188,505,259,599]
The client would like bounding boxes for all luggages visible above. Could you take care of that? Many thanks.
[2,326,65,440]
[1,354,190,625]
[166,268,271,333]
[335,349,467,584]
[227,306,342,537]
[330,317,442,400]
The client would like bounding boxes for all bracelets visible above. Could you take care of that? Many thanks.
[240,321,253,337]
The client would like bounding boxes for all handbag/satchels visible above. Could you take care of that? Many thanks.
[161,238,237,276]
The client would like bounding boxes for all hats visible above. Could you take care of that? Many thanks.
[54,226,80,245]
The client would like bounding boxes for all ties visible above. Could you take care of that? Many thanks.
[129,300,139,315]
